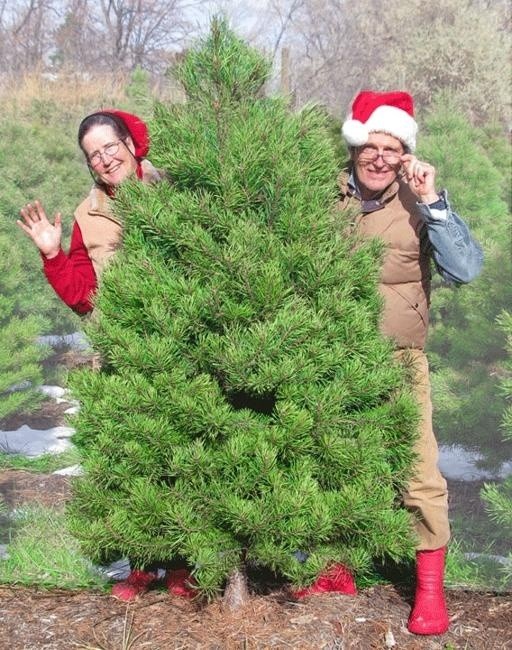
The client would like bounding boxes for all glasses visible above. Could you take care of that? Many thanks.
[89,141,122,167]
[360,150,403,165]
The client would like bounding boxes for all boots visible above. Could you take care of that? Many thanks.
[409,546,448,635]
[293,560,355,600]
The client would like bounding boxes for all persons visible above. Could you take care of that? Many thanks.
[17,108,200,604]
[288,89,485,634]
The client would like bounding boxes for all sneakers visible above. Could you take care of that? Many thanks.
[110,571,157,604]
[168,570,201,597]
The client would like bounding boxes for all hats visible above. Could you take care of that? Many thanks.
[342,92,418,152]
[78,110,150,158]
